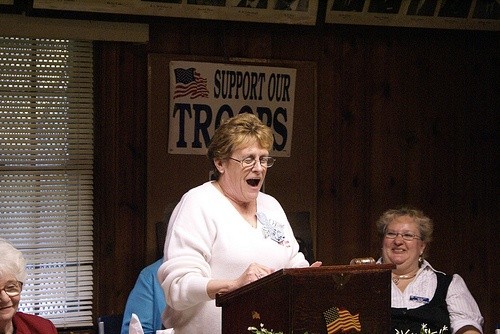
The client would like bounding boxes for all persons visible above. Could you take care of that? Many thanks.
[156,112,310,334]
[121,258,167,334]
[376,207,485,334]
[0,239,57,334]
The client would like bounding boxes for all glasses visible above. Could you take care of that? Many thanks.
[0,279,24,297]
[385,230,423,241]
[228,155,277,170]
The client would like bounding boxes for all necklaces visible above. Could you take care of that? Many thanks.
[391,274,416,285]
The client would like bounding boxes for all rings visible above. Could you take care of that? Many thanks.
[258,273,263,279]
